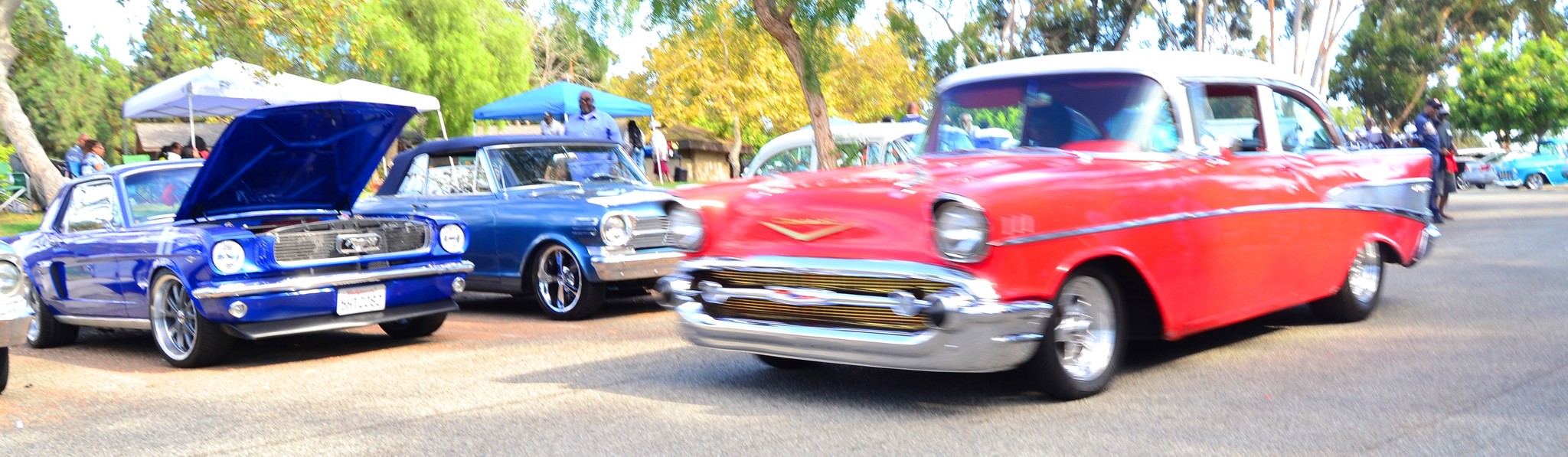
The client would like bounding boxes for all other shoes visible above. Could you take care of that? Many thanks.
[1434,213,1444,223]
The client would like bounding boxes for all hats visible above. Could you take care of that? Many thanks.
[1438,101,1452,116]
[1427,97,1442,109]
[198,150,210,160]
[648,121,660,129]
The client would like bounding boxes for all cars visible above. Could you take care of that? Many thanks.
[658,50,1441,399]
[0,241,36,395]
[348,137,686,321]
[21,101,472,369]
[1450,138,1568,190]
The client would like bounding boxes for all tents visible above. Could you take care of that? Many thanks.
[123,57,456,179]
[473,81,665,186]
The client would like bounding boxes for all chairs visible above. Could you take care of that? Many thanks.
[0,164,32,214]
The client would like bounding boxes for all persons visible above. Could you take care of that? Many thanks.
[1414,96,1457,222]
[159,146,169,160]
[565,92,623,181]
[648,120,675,184]
[189,134,209,159]
[899,101,928,143]
[64,134,109,178]
[958,113,981,145]
[167,142,183,160]
[540,111,565,137]
[1026,102,1072,149]
[181,145,199,158]
[1354,115,1416,147]
[869,115,898,164]
[624,120,647,177]
